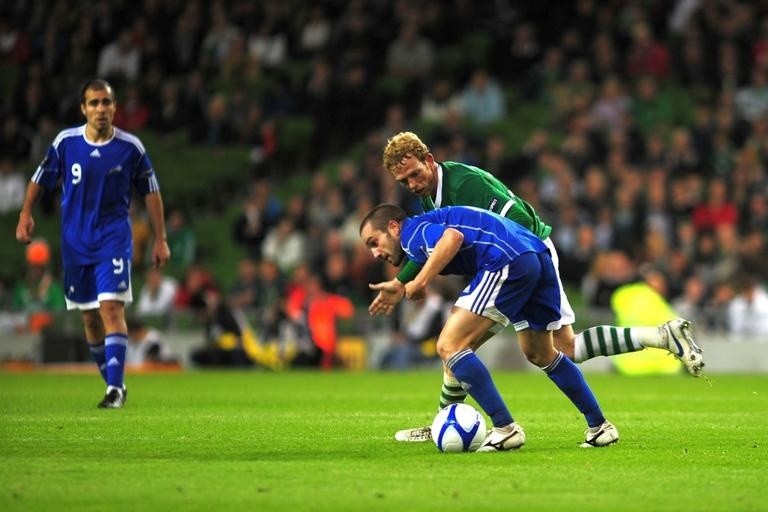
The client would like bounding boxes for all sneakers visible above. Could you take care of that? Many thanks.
[393,424,433,443]
[662,315,706,378]
[578,420,620,450]
[95,382,128,410]
[475,424,527,454]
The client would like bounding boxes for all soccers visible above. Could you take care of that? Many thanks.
[431,402,487,453]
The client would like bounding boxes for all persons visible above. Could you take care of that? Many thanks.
[18,77,170,409]
[382,130,705,443]
[360,202,620,452]
